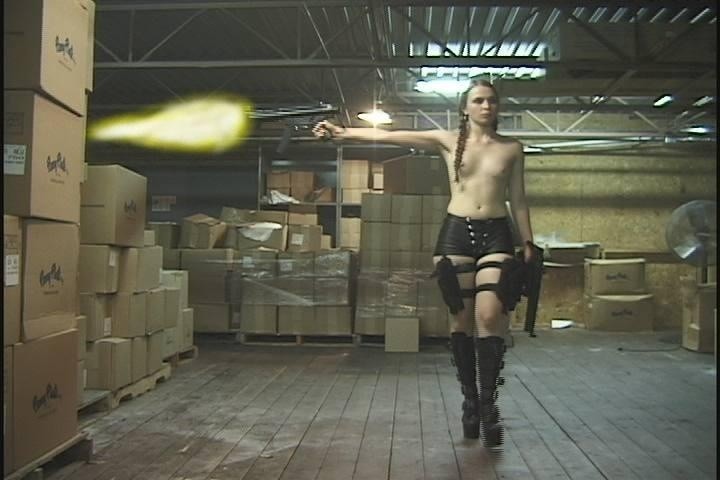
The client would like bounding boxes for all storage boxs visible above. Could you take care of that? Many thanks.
[582,256,655,335]
[679,288,716,353]
[0,0,452,479]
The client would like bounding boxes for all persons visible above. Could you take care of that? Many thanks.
[310,80,535,447]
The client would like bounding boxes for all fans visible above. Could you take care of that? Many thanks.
[664,199,717,285]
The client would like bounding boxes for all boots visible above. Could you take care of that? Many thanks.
[450,331,480,437]
[476,336,506,448]
